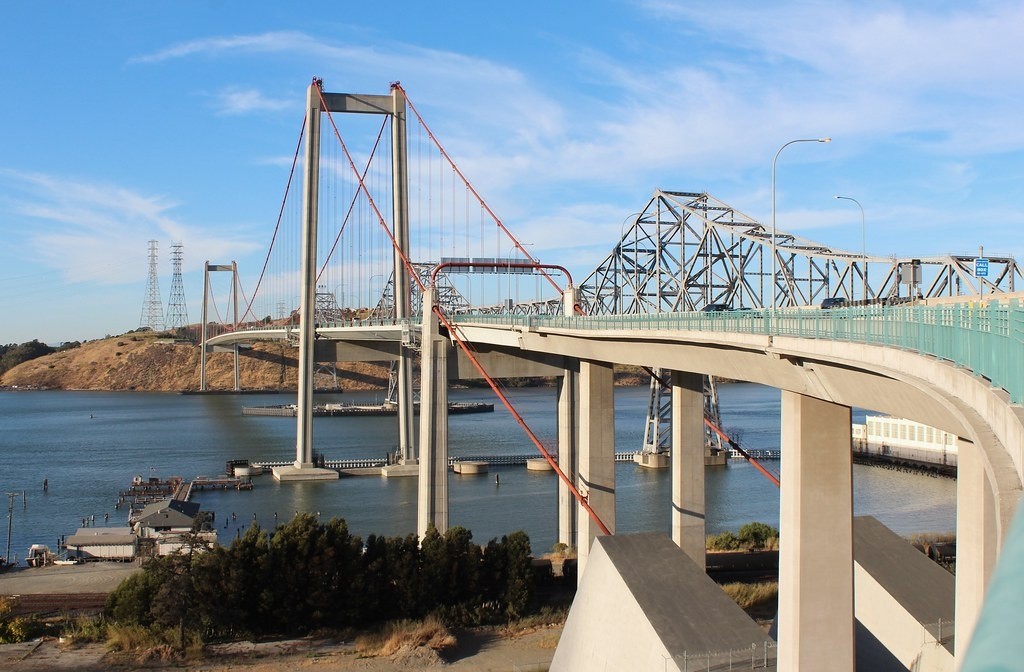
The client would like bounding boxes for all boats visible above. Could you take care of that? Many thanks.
[23,542,56,567]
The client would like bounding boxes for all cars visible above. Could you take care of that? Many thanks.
[704,303,728,318]
[820,297,853,309]
[737,308,762,319]
[722,308,740,320]
